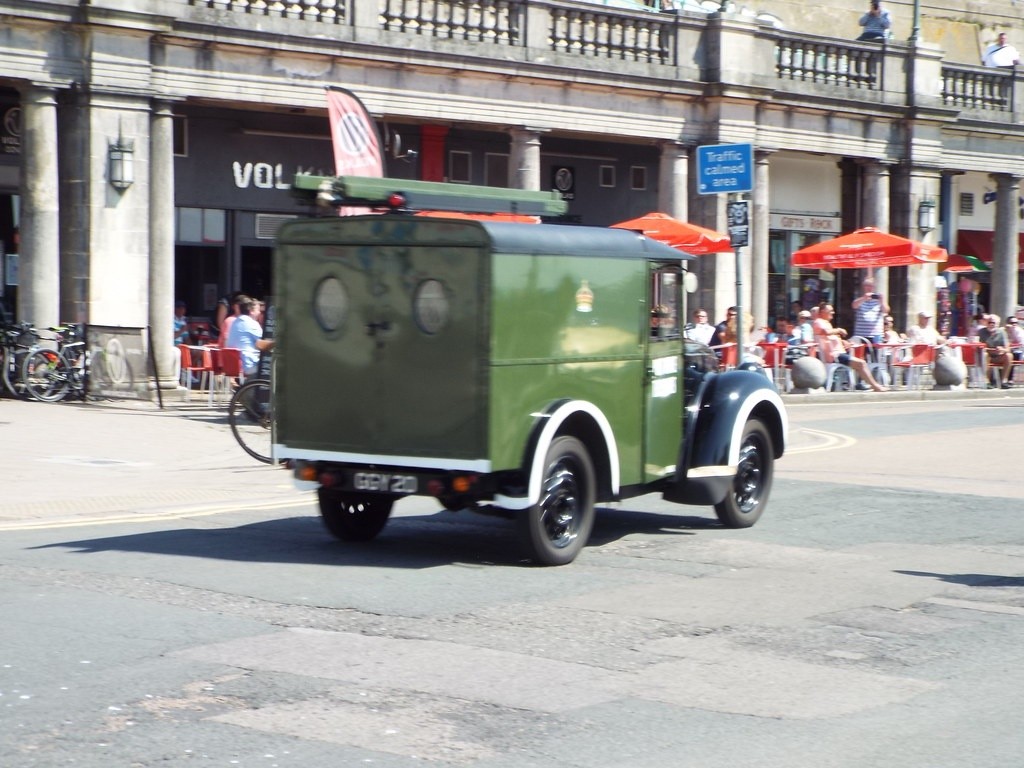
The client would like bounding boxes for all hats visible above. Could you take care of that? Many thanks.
[918,312,931,320]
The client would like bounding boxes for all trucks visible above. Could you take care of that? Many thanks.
[270,173,787,565]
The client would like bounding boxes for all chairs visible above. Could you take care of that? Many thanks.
[177,321,245,406]
[706,331,1023,392]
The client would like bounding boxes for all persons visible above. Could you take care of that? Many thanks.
[856,0,891,42]
[684,308,721,355]
[745,278,907,391]
[216,291,278,389]
[938,253,992,274]
[907,311,946,346]
[978,314,1024,388]
[717,307,738,351]
[982,33,1021,97]
[175,299,199,383]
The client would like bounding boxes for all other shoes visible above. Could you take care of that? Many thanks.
[1001,383,1011,389]
[987,381,997,388]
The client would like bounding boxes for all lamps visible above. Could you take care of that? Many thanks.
[920,197,937,232]
[107,134,135,187]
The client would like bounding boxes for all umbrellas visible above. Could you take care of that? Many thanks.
[609,211,737,306]
[792,227,949,277]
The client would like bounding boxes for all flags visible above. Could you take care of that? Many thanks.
[326,85,387,216]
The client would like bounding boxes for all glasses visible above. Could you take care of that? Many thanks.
[988,321,997,325]
[728,313,738,317]
[1008,321,1018,325]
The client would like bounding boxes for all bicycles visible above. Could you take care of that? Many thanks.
[230,381,288,464]
[0,320,135,402]
